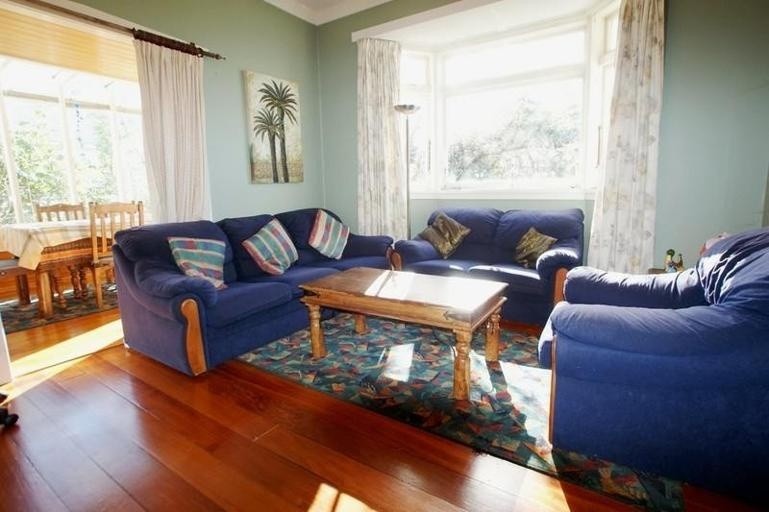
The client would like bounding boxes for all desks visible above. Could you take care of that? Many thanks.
[648,266,671,276]
[0,215,146,316]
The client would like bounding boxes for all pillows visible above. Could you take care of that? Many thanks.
[168,233,230,290]
[240,214,298,276]
[421,209,471,260]
[514,224,558,270]
[306,207,349,258]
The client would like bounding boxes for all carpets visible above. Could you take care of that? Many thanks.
[0,282,120,334]
[235,312,690,511]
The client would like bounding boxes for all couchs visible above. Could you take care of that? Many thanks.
[394,199,584,332]
[535,226,769,477]
[106,203,392,376]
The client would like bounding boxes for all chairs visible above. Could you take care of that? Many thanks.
[80,197,145,307]
[33,198,89,312]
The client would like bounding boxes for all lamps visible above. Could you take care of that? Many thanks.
[393,100,422,241]
